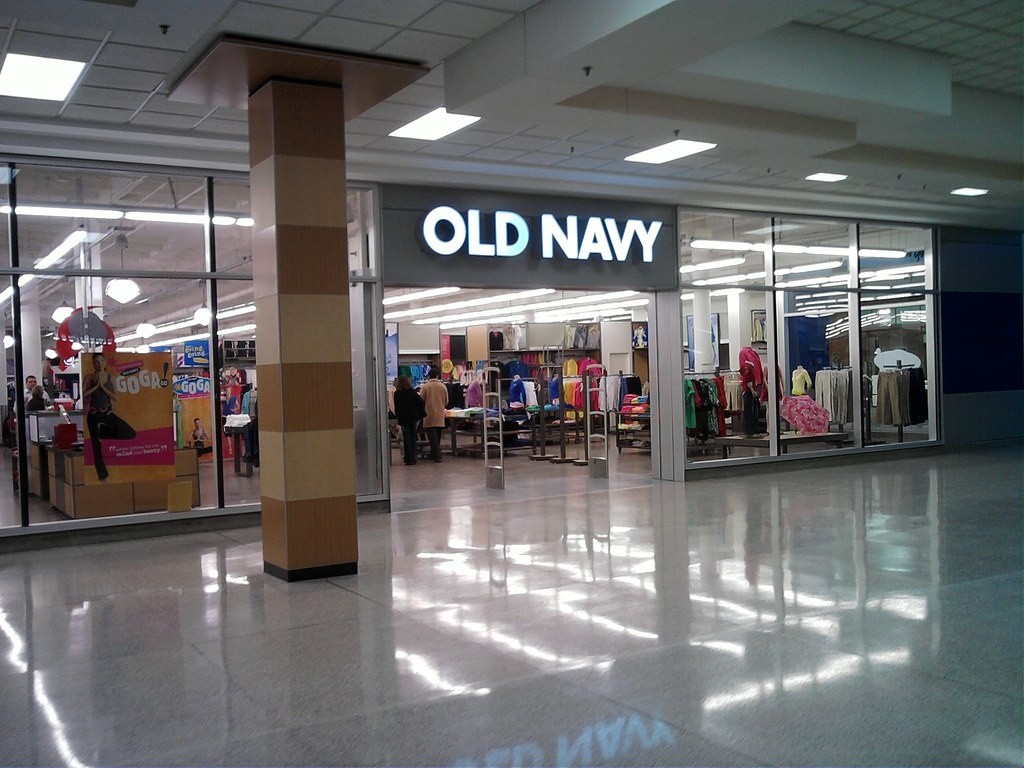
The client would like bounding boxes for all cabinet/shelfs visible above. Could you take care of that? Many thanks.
[45,440,201,519]
[30,441,52,498]
[615,412,719,454]
[221,339,256,360]
[388,406,580,466]
[487,348,603,432]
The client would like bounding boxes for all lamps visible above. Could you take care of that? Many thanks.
[0,162,254,360]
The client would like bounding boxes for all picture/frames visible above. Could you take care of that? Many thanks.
[750,309,767,343]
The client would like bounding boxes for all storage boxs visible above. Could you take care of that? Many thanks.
[29,409,83,444]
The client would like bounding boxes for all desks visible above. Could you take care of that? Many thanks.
[714,429,849,459]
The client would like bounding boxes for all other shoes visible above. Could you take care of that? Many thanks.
[404,461,415,465]
[436,459,441,462]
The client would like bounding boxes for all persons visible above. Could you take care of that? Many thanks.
[13,376,51,413]
[393,375,426,465]
[739,346,769,438]
[466,376,482,407]
[549,373,560,424]
[791,365,812,396]
[420,369,448,463]
[509,374,527,409]
[188,418,213,457]
[82,352,136,481]
